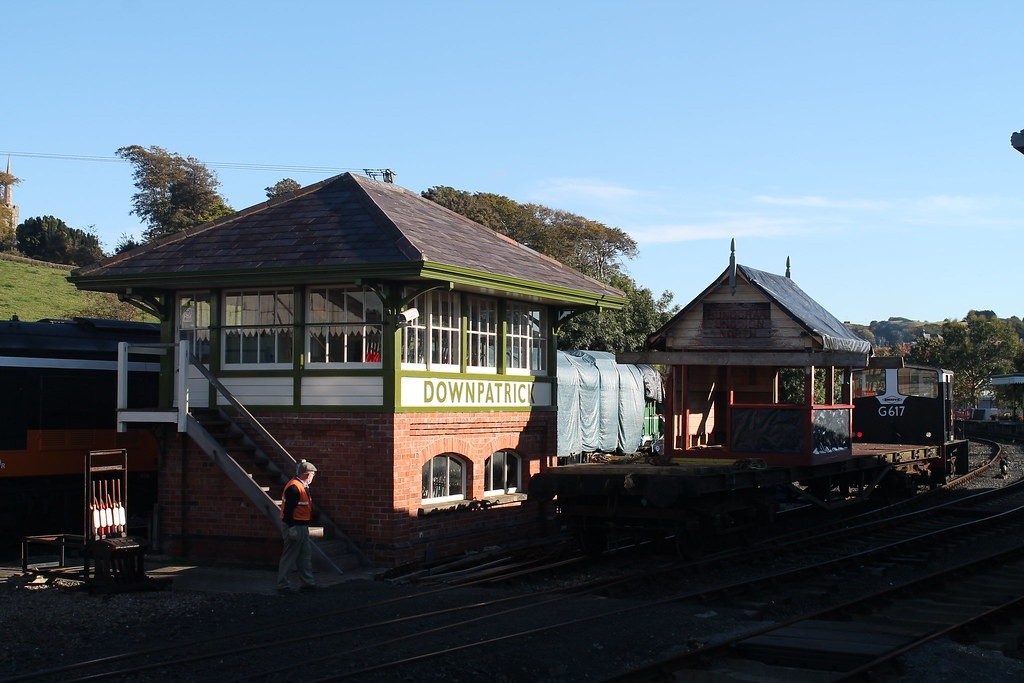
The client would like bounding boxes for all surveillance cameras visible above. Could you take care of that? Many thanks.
[398,307,420,323]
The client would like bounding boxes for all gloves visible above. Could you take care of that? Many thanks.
[289,526,297,536]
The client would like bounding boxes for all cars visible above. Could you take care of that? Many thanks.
[952,406,1024,423]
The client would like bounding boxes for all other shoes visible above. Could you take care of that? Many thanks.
[280,588,296,596]
[301,584,328,593]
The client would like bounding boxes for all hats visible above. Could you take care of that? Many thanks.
[297,459,318,472]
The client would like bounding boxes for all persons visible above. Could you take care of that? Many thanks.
[276,462,320,594]
